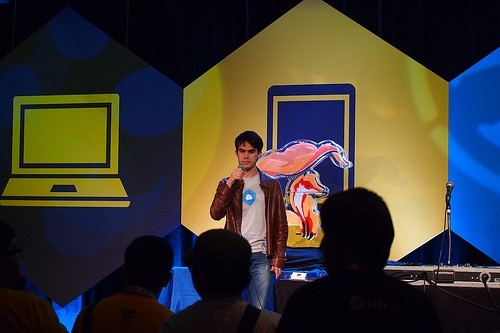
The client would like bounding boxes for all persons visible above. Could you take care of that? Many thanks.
[209,131,288,310]
[70,235,175,333]
[162,229,281,333]
[276,188,441,333]
[0,221,70,332]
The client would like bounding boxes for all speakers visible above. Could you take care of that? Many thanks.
[418,285,500,333]
[273,280,311,314]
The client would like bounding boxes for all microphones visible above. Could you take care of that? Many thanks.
[233,164,244,190]
[446,182,454,203]
